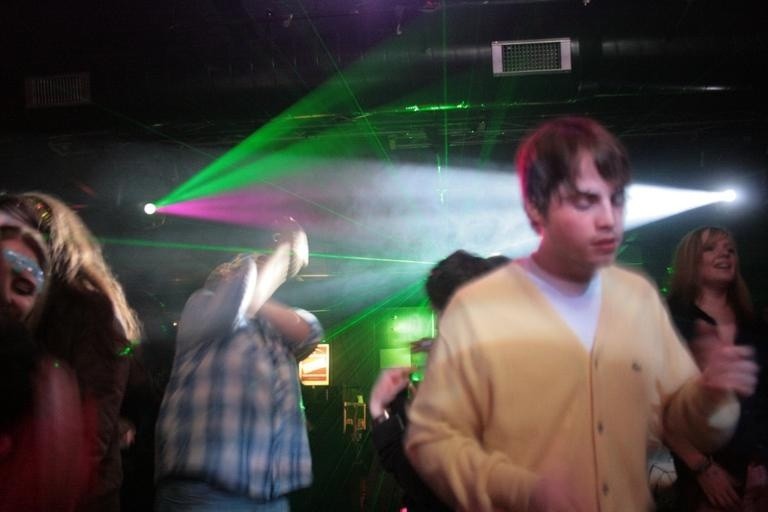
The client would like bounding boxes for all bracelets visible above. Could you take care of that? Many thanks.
[373,409,390,425]
[693,454,714,478]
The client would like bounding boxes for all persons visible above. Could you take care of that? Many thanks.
[365,248,508,512]
[150,216,324,512]
[0,210,96,510]
[401,119,760,510]
[660,225,768,510]
[14,185,143,511]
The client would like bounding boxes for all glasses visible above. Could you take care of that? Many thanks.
[0,192,55,230]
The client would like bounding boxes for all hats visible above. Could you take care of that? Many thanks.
[422,251,508,306]
